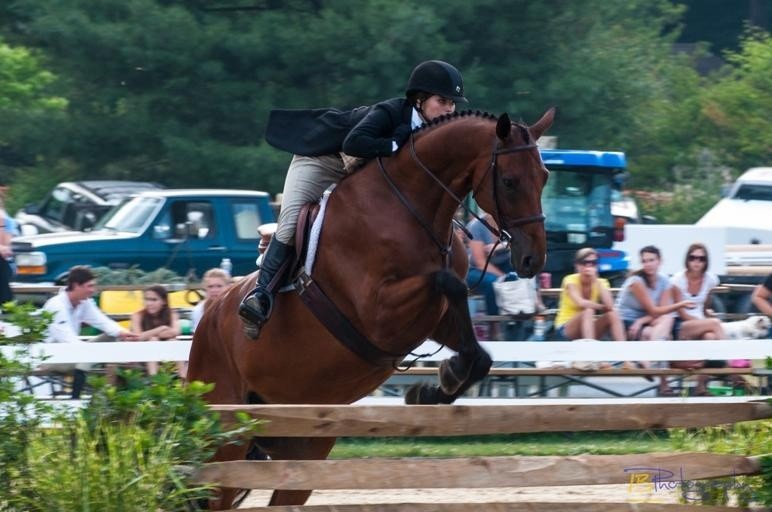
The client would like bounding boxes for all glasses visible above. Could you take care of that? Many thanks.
[687,254,708,264]
[578,258,600,265]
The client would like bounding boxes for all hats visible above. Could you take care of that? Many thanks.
[407,59,469,104]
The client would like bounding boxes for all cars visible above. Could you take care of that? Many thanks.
[17,181,167,236]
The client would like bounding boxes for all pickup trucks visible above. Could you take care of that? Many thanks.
[11,190,274,304]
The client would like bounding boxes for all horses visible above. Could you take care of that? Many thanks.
[185,108,560,511]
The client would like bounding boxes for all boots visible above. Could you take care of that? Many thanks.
[238,231,293,341]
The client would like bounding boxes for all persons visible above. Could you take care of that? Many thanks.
[751,272,772,396]
[128,284,187,390]
[464,211,549,341]
[553,248,628,369]
[669,242,727,369]
[190,267,231,336]
[237,59,469,325]
[0,172,21,317]
[38,266,141,400]
[613,246,698,396]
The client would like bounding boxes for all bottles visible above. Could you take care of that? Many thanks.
[221,257,231,280]
[534,317,546,340]
